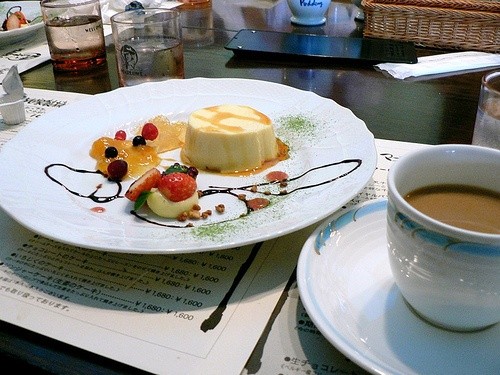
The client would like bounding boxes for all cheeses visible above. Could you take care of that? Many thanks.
[184,102,278,173]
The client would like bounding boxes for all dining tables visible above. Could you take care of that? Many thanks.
[0,0,500,375]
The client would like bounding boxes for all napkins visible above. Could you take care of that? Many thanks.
[376,50,500,80]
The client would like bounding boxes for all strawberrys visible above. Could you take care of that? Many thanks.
[4,11,27,30]
[159,172,197,202]
[125,168,161,200]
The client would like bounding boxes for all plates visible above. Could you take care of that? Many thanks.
[0,0,70,45]
[0,76,377,257]
[297,199,499,375]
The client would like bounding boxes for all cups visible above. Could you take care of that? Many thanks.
[471,68,500,151]
[161,0,217,49]
[287,0,331,25]
[386,142,500,334]
[39,0,108,77]
[110,7,185,88]
